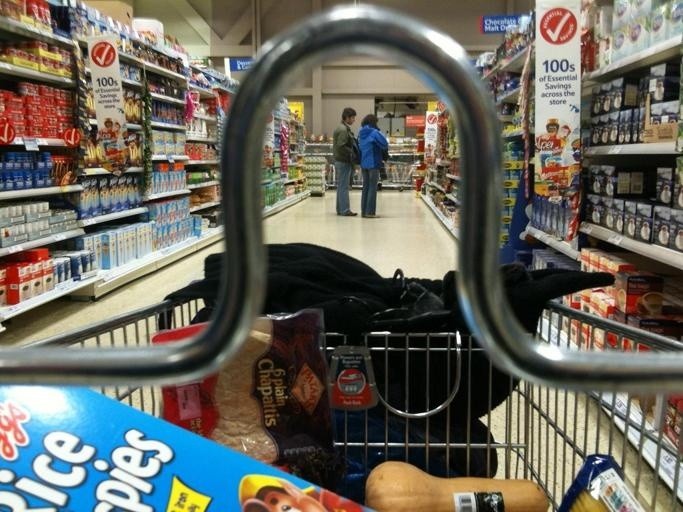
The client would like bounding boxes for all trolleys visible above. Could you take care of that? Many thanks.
[0,6,681,511]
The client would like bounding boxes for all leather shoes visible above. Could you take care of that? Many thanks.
[337,210,358,216]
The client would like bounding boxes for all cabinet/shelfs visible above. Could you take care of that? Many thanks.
[0,1,327,331]
[419,0,683,502]
[327,143,418,189]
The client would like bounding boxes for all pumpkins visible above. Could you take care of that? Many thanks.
[366,460,548,512]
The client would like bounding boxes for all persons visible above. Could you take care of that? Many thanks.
[332,106,358,216]
[355,113,389,218]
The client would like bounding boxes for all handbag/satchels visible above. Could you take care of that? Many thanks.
[351,136,362,165]
[159,243,615,480]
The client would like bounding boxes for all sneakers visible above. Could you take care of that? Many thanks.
[362,212,380,219]
